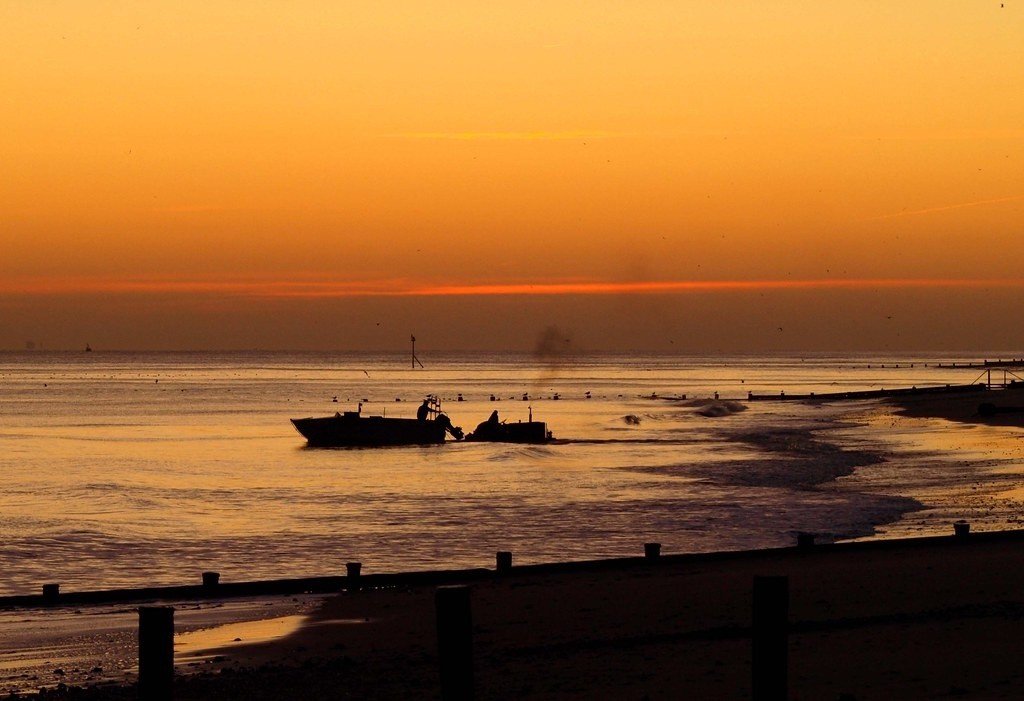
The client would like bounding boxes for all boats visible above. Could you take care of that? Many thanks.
[289,413,446,447]
[471,421,567,444]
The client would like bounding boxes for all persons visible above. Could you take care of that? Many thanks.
[417,400,442,420]
